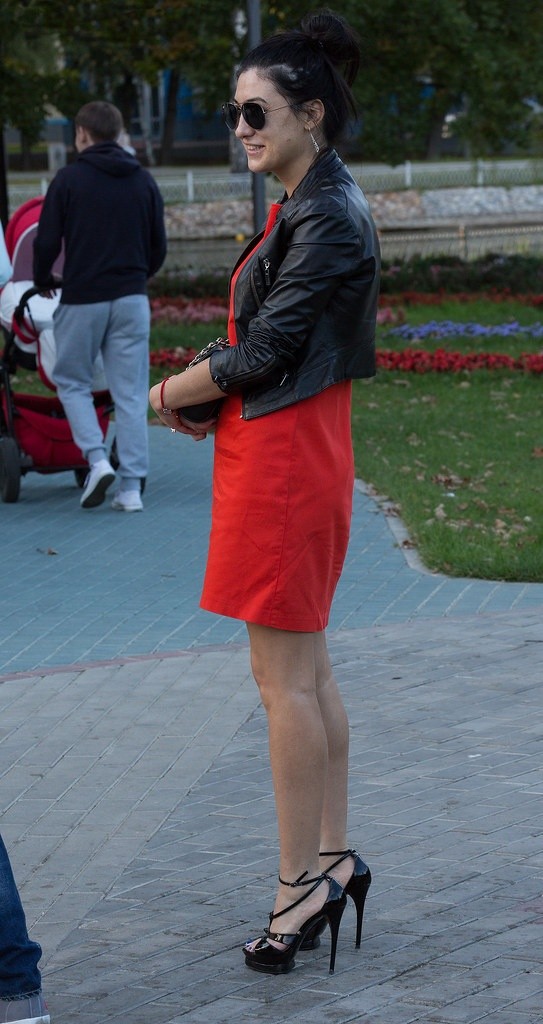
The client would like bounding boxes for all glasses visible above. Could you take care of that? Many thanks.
[221,103,304,131]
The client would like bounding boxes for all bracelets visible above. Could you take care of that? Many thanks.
[161,379,173,415]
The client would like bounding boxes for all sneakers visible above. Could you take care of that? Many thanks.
[111,491,143,513]
[80,459,116,508]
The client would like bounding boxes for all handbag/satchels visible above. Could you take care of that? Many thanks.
[176,337,231,432]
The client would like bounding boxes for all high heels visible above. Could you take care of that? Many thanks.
[298,849,371,951]
[242,872,348,975]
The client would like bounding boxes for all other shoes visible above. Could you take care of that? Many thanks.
[0,994,50,1024]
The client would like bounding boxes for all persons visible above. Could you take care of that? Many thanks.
[31,100,168,512]
[0,836,50,1024]
[148,14,380,975]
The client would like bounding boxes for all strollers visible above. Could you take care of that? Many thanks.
[0,195,116,503]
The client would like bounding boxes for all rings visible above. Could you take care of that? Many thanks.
[171,428,176,433]
